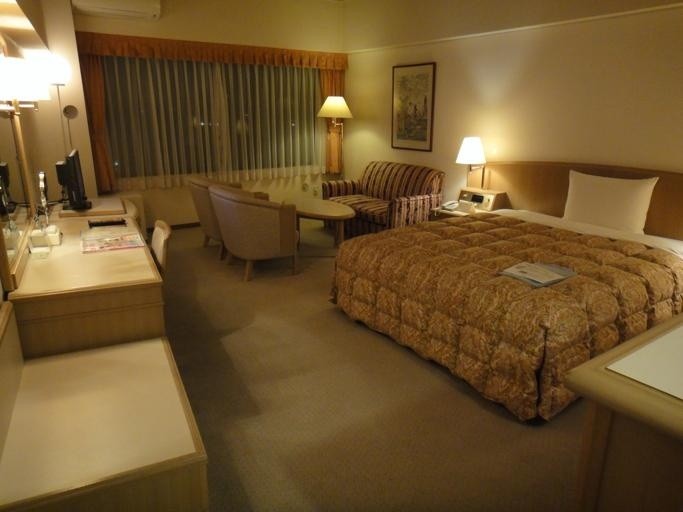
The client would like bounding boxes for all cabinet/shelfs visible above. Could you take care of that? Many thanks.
[0,298,210,512]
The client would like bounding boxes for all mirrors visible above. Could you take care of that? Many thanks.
[1,34,37,294]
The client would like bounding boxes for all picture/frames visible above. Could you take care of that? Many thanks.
[391,61,437,152]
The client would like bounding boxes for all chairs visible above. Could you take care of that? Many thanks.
[149,219,171,285]
[209,183,299,283]
[187,173,266,260]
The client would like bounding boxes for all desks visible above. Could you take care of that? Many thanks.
[567,311,683,512]
[116,191,148,237]
[283,196,355,248]
[9,194,165,361]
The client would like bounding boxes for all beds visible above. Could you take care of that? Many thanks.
[328,159,683,422]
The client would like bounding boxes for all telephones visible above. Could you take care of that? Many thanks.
[440,201,458,212]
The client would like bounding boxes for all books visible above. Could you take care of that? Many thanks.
[499,261,576,288]
[79,224,144,254]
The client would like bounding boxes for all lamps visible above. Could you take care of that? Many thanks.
[0,56,50,114]
[454,137,488,189]
[316,95,355,161]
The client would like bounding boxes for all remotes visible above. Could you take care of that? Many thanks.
[88,217,126,228]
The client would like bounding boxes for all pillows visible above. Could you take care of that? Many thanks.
[563,170,659,235]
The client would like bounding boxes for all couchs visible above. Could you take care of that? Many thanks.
[322,161,446,238]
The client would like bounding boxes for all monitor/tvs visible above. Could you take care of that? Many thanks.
[56,148,91,210]
[0,161,16,216]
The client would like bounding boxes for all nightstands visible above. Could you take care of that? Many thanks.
[430,188,505,222]
